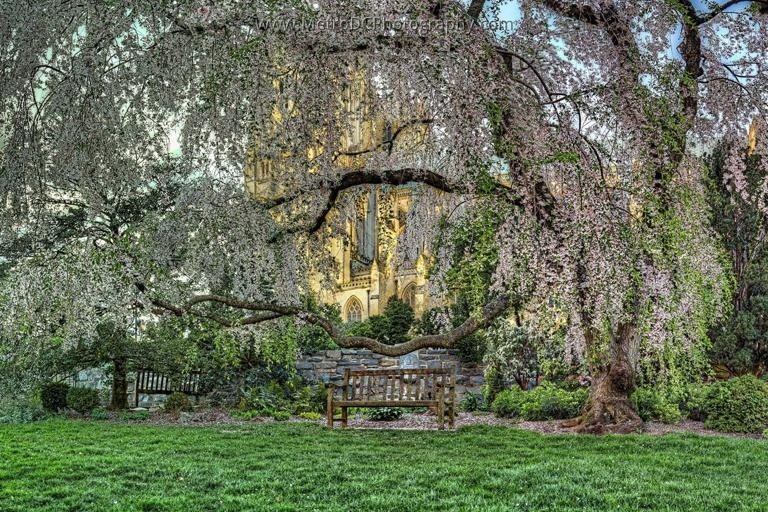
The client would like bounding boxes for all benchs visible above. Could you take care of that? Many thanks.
[326,364,456,430]
[135,366,208,407]
